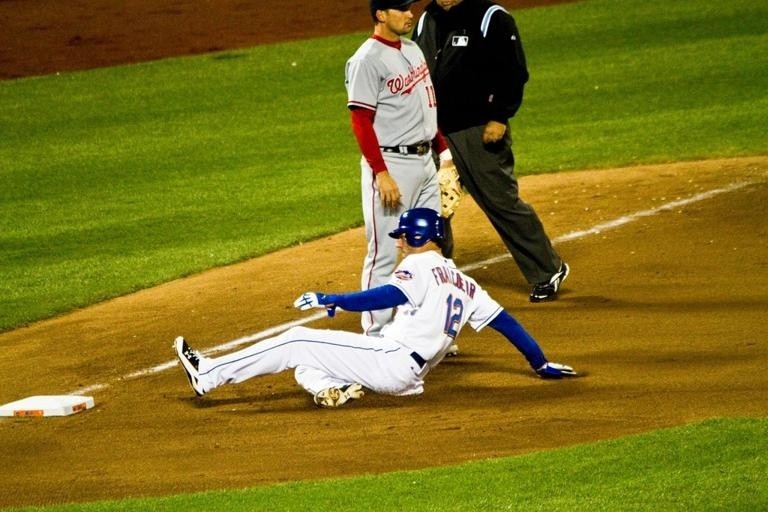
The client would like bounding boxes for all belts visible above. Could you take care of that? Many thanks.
[383,141,431,156]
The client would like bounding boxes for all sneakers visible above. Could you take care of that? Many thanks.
[172,335,207,397]
[530,261,571,302]
[312,383,365,409]
[446,343,459,356]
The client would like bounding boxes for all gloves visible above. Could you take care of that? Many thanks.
[535,361,577,380]
[293,291,338,311]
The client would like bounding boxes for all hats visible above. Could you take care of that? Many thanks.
[369,1,421,14]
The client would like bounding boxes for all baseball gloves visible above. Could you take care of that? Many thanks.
[438,165,464,219]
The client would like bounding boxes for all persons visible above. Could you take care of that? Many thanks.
[341,0,462,361]
[409,0,569,304]
[169,206,581,410]
